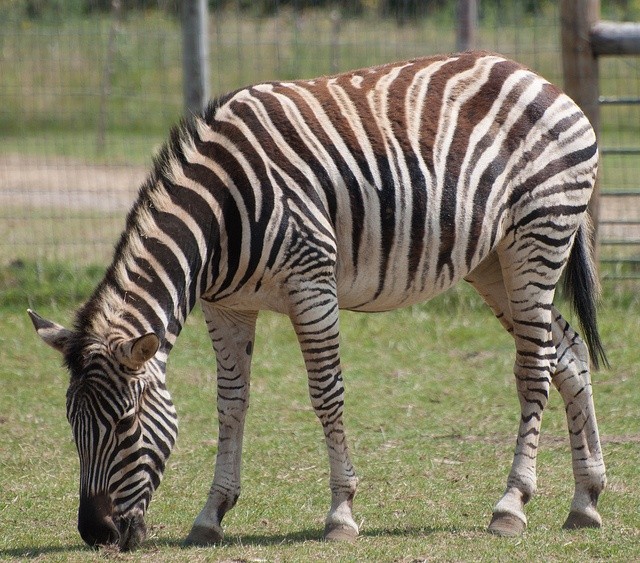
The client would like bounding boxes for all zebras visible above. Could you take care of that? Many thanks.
[27,50,611,552]
